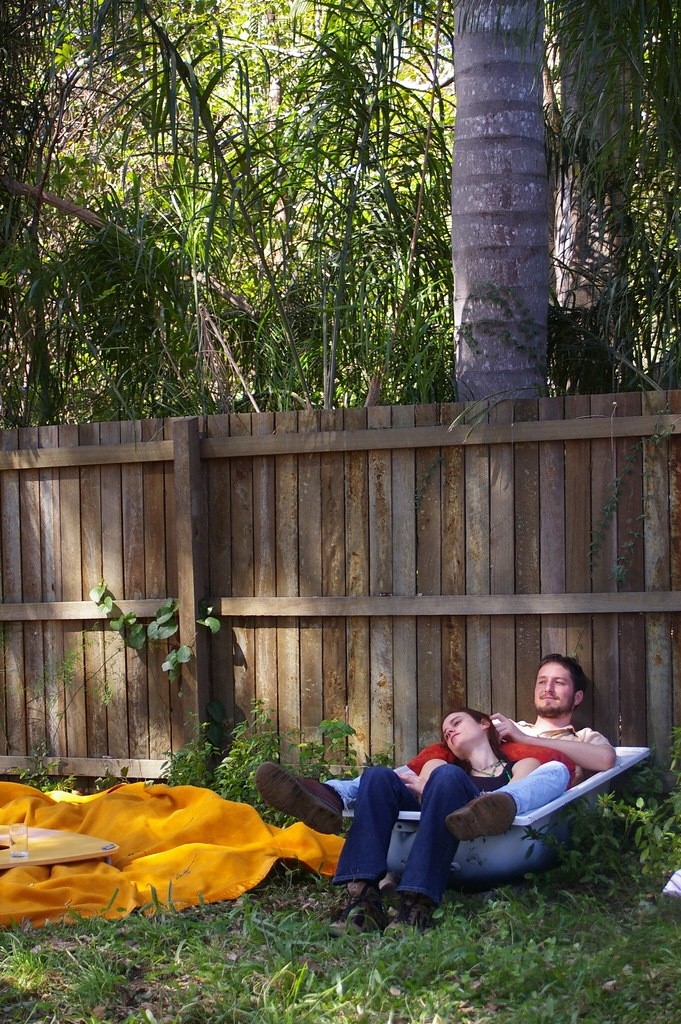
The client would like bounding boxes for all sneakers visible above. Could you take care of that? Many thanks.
[256,761,343,835]
[383,891,435,941]
[329,879,387,939]
[446,791,517,841]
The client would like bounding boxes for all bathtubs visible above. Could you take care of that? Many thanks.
[343,746,650,888]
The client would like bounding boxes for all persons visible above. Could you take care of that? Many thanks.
[256,653,616,835]
[327,706,540,937]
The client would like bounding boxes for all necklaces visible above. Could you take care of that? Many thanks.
[474,759,512,782]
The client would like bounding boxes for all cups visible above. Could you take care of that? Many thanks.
[9,823,29,857]
